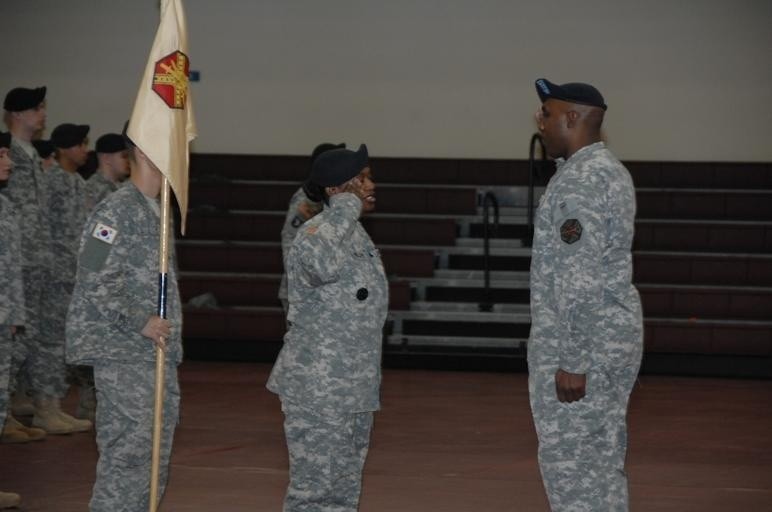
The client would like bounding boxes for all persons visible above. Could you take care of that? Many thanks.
[277,142,347,320]
[0,85,132,509]
[265,148,389,512]
[527,78,645,512]
[64,119,184,512]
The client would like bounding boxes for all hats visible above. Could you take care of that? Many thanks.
[0,86,129,153]
[308,143,369,189]
[536,77,607,109]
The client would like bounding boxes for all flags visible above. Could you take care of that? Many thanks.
[126,0,198,235]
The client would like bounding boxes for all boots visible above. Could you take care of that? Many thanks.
[0,491,21,507]
[1,382,97,442]
